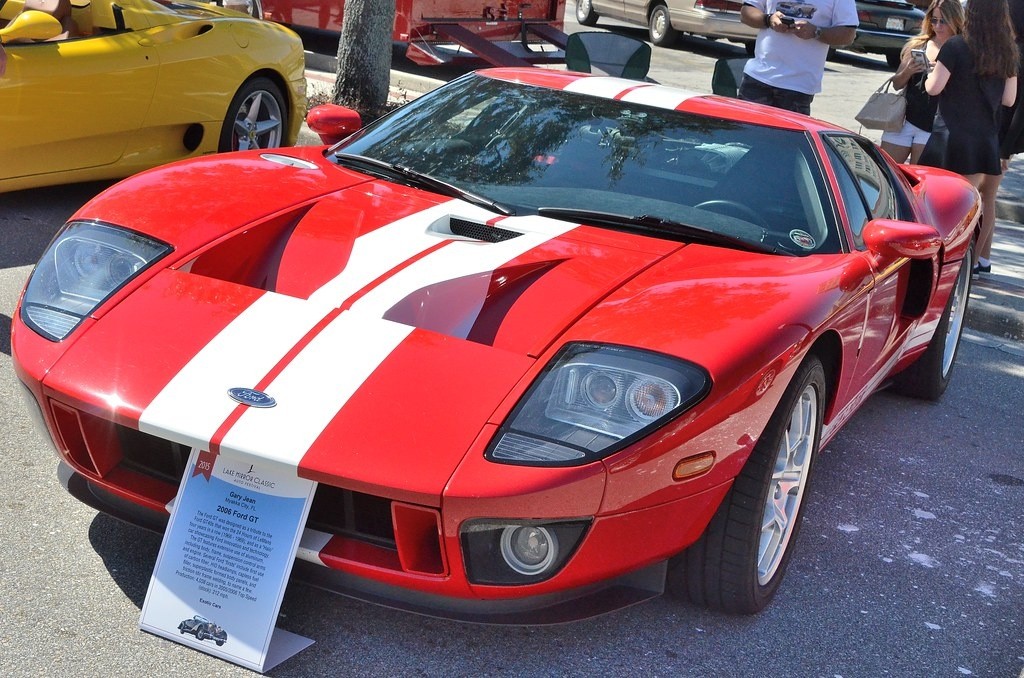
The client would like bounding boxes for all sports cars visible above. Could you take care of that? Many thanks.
[0,0,310,193]
[10,66,983,627]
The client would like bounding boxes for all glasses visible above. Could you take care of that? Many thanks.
[929,17,947,24]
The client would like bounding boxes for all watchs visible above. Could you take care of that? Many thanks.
[814,25,823,41]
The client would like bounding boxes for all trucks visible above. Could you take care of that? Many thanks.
[203,0,573,69]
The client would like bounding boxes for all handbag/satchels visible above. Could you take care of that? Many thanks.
[856,75,907,133]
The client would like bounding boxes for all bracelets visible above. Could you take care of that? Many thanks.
[763,13,772,28]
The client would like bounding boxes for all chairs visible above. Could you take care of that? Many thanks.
[704,137,811,246]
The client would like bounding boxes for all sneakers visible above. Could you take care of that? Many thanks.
[977,258,992,272]
[970,264,980,279]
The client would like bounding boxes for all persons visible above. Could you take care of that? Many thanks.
[879,1,965,166]
[969,0,1024,279]
[735,0,860,117]
[915,0,1018,188]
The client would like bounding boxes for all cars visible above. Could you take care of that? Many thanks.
[826,0,926,65]
[574,0,759,57]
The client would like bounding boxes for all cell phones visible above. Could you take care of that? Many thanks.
[781,16,795,28]
[911,49,928,70]
